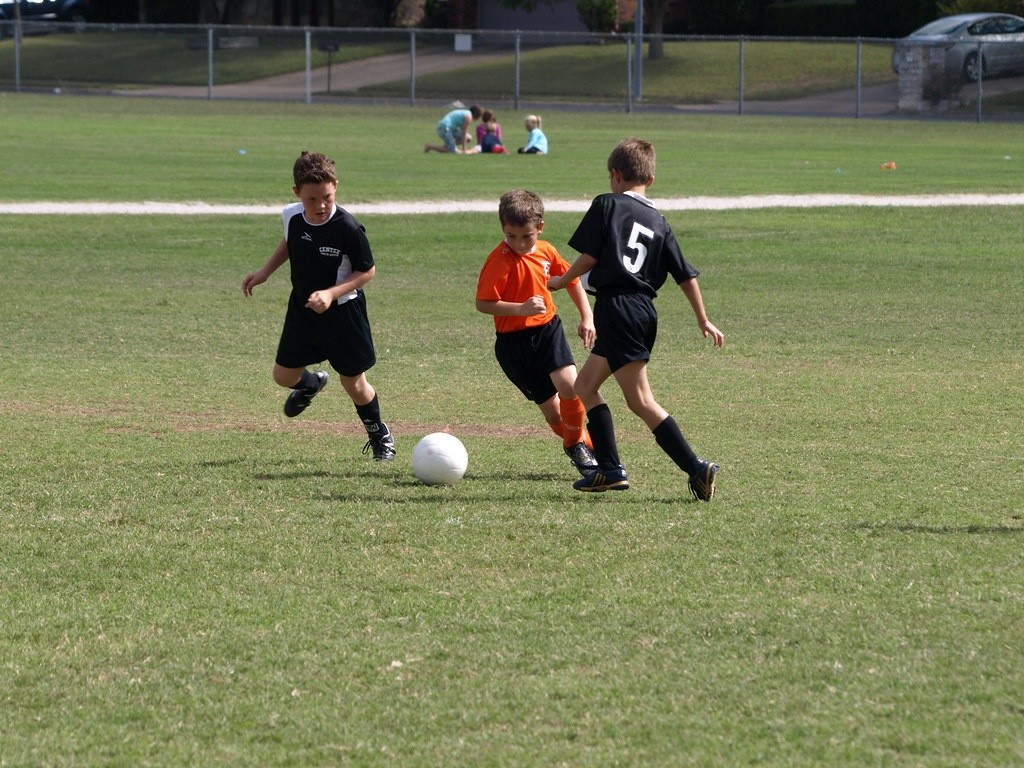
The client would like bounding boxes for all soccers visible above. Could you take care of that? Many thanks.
[411,432,469,485]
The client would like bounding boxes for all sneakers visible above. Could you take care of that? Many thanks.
[362,422,396,460]
[285,370,328,417]
[563,442,599,477]
[688,459,720,502]
[573,463,630,491]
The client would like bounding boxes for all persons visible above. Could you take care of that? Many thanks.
[241,151,397,464]
[475,188,597,479]
[545,137,724,503]
[615,0,647,42]
[424,105,548,158]
